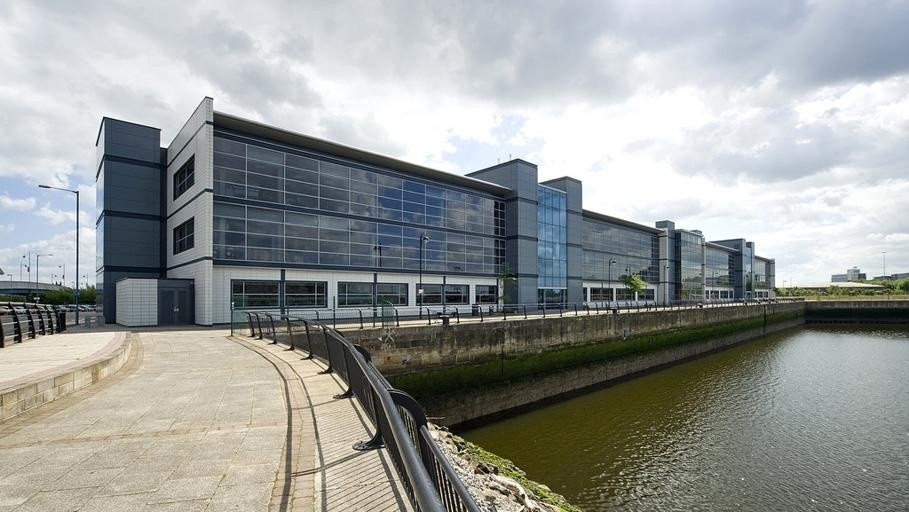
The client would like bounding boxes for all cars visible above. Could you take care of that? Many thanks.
[1,302,96,315]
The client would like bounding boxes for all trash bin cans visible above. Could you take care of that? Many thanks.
[59,312,66,332]
[472,303,478,316]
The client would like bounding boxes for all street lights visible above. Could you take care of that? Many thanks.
[39,183,81,325]
[418,231,430,317]
[496,276,517,313]
[624,264,631,279]
[35,253,53,303]
[608,256,616,309]
[712,267,720,306]
[22,250,30,282]
[83,272,88,289]
[664,263,672,305]
[58,264,66,285]
[881,251,887,276]
[783,279,786,298]
[7,272,13,303]
[372,239,384,267]
